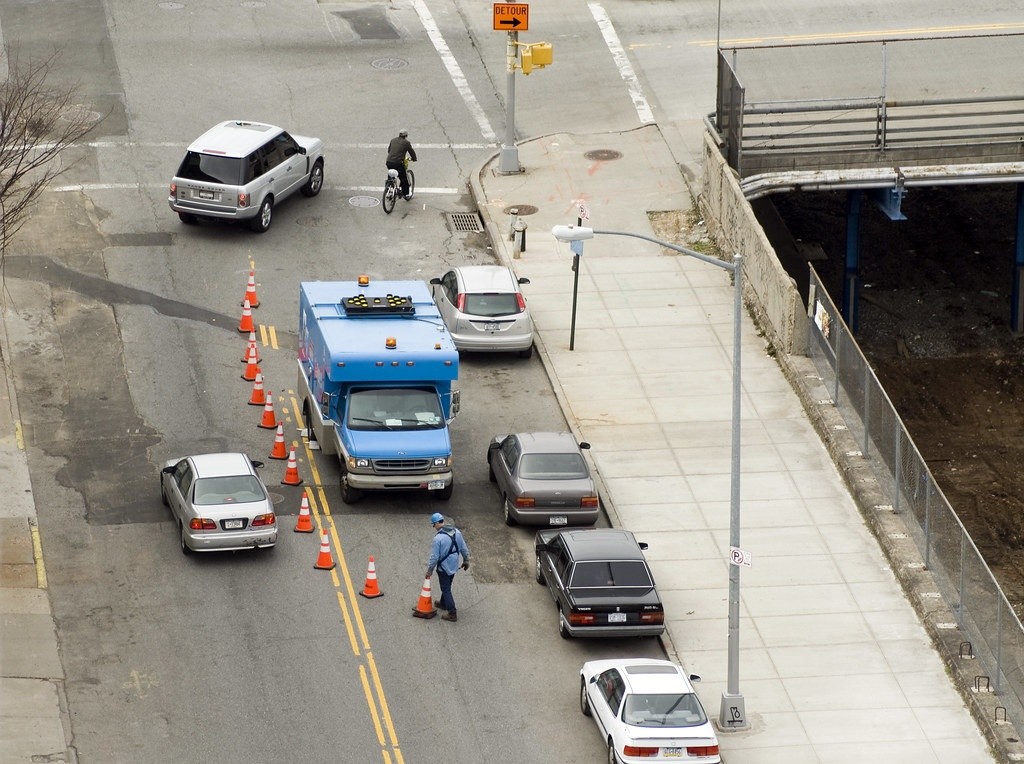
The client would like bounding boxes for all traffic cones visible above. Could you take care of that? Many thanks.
[257,390,278,429]
[293,492,315,533]
[359,556,384,598]
[268,422,291,459]
[241,344,263,381]
[238,271,262,364]
[248,373,267,405]
[410,577,438,618]
[280,446,304,486]
[313,528,336,570]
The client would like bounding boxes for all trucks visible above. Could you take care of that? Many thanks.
[297,275,462,504]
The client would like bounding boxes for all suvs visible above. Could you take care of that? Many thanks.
[169,120,326,232]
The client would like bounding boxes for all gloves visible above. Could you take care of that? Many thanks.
[461,559,470,571]
[425,569,433,579]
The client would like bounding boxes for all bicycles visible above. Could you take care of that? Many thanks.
[382,158,415,215]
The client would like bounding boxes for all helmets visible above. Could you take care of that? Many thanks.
[430,512,444,524]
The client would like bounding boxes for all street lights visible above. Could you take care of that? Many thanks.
[551,224,746,736]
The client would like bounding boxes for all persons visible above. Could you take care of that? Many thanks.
[425,513,470,622]
[386,128,417,199]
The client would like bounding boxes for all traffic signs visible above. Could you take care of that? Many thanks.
[494,4,530,30]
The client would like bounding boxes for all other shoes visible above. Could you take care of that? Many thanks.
[434,600,447,610]
[405,192,412,197]
[441,614,457,621]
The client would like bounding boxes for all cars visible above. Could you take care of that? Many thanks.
[430,265,535,361]
[581,659,725,763]
[533,527,665,641]
[160,451,278,555]
[488,430,600,531]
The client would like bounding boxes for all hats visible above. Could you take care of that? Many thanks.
[398,129,409,137]
